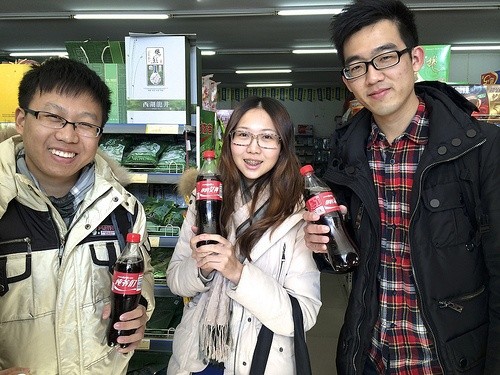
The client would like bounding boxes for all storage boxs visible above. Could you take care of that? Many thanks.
[0,63,42,122]
[63,30,202,125]
[297,125,313,134]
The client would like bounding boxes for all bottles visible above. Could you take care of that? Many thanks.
[107,233,144,349]
[195,150,223,248]
[300,165,361,272]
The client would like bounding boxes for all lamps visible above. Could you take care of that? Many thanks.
[9,53,69,57]
[291,49,337,54]
[70,14,175,19]
[275,8,345,16]
[246,83,293,87]
[235,70,292,73]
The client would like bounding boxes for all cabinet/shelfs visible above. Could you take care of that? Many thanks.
[0,106,223,375]
[295,133,331,175]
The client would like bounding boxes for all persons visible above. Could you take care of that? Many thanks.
[302,0,500,375]
[0,57,156,375]
[167,97,322,375]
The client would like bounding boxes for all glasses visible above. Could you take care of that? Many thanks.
[229,129,282,149]
[340,45,411,82]
[22,107,103,139]
